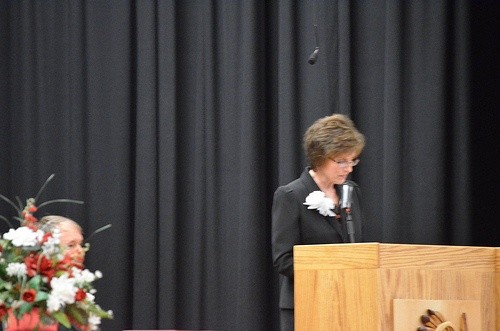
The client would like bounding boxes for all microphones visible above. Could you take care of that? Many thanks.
[341,180,354,210]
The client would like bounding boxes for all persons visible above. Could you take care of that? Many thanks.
[271,113,366,331]
[40,215,85,263]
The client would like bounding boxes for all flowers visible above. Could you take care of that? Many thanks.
[0,174,114,331]
[303,190,337,217]
